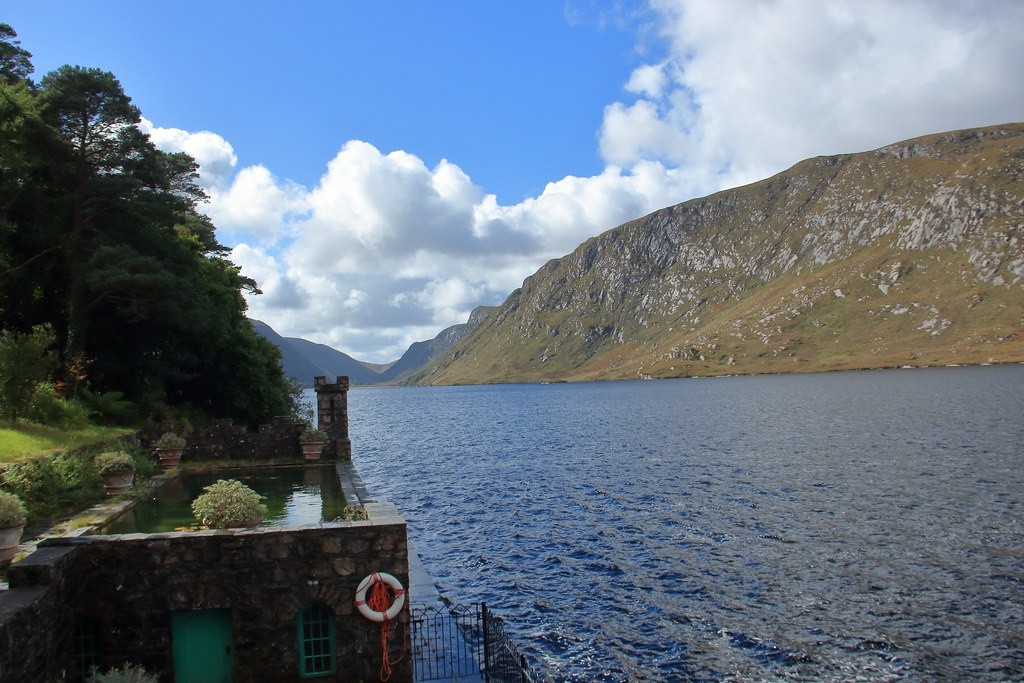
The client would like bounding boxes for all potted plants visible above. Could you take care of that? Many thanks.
[137,407,306,457]
[155,432,186,470]
[95,451,136,498]
[0,490,31,561]
[191,478,269,532]
[298,427,329,460]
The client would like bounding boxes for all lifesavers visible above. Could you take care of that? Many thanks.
[355,572,406,621]
